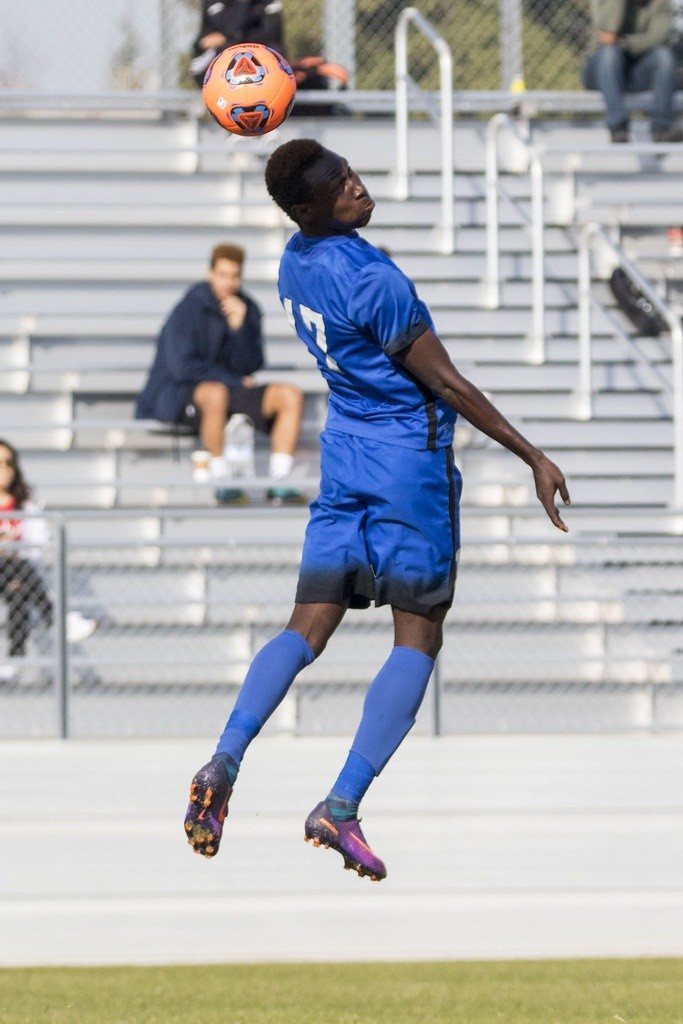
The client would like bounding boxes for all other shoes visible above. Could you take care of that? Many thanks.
[266,485,308,506]
[2,663,40,689]
[609,124,629,142]
[51,610,96,645]
[652,127,673,142]
[213,486,250,504]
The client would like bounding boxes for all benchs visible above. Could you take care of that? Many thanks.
[0,90,683,738]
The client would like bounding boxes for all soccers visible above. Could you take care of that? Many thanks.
[201,43,298,136]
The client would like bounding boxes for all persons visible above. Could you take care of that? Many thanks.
[188,0,329,118]
[183,138,574,884]
[0,438,97,658]
[581,0,683,142]
[134,244,310,507]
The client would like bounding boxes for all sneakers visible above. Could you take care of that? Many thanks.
[182,758,235,860]
[304,801,388,882]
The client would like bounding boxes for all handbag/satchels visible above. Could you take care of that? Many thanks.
[225,412,258,477]
